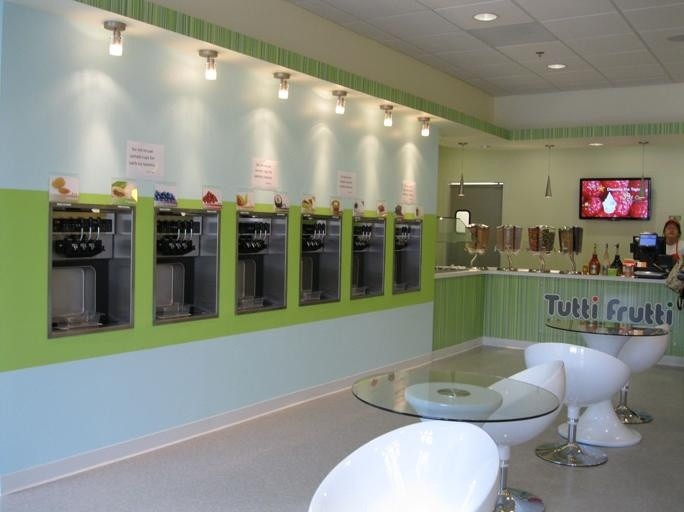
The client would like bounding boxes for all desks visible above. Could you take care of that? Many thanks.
[545,319,672,448]
[349,365,561,427]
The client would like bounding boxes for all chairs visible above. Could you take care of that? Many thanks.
[482,359,567,512]
[305,416,503,510]
[522,343,630,468]
[613,323,670,425]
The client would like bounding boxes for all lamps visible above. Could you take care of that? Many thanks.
[638,140,650,197]
[380,105,393,127]
[101,20,128,58]
[455,142,468,196]
[330,89,348,115]
[542,144,555,198]
[273,71,291,100]
[416,115,431,137]
[197,49,218,81]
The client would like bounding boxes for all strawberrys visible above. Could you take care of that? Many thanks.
[582,181,647,218]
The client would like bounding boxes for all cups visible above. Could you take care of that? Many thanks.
[623,266,634,279]
[607,267,618,276]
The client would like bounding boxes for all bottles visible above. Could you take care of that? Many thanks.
[600,241,610,274]
[589,242,600,275]
[582,263,588,276]
[611,244,623,275]
[464,222,584,254]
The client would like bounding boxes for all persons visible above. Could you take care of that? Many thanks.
[661,219,684,272]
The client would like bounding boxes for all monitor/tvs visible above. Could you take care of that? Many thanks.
[579,178,651,220]
[633,236,666,271]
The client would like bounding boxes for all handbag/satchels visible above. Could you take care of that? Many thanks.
[664,258,684,298]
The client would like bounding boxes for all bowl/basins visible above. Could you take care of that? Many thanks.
[56,186,71,197]
